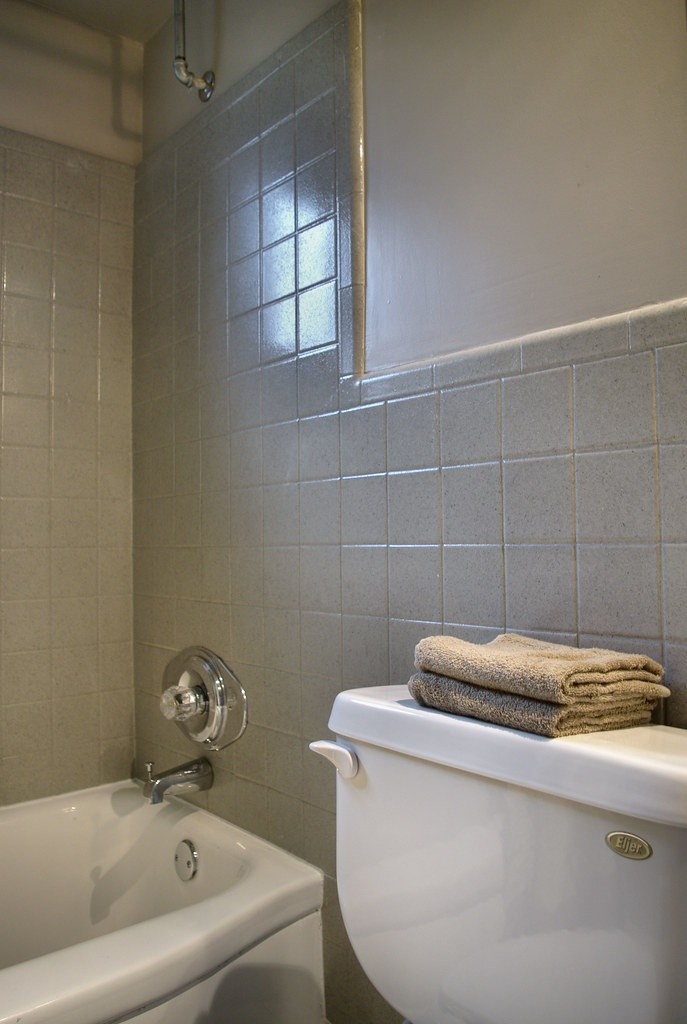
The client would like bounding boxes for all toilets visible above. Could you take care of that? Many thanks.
[309,676,687,1023]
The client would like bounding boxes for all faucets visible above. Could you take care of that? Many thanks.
[141,755,213,806]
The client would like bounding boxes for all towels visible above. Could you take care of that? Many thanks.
[405,629,671,741]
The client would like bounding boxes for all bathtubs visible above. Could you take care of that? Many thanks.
[1,774,329,1024]
